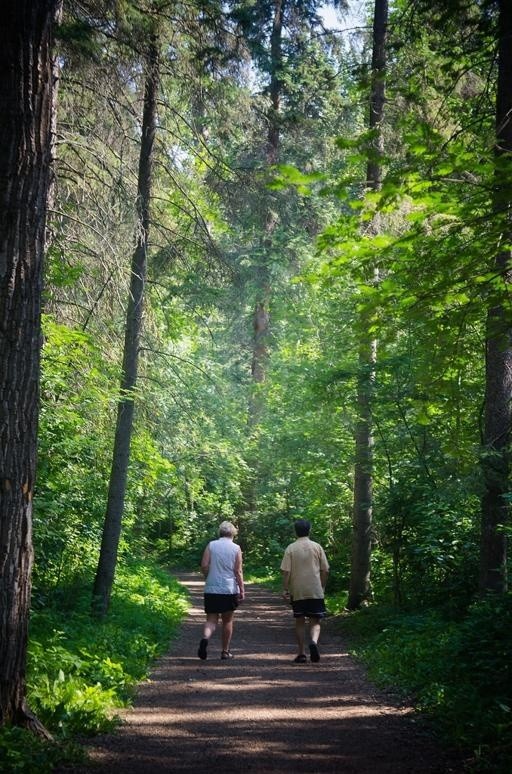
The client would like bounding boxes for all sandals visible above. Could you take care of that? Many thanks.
[221,650,234,659]
[198,639,208,660]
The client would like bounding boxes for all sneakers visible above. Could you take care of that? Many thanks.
[292,655,307,663]
[308,642,321,663]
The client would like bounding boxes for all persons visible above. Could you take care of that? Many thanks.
[280,519,330,663]
[198,521,245,659]
